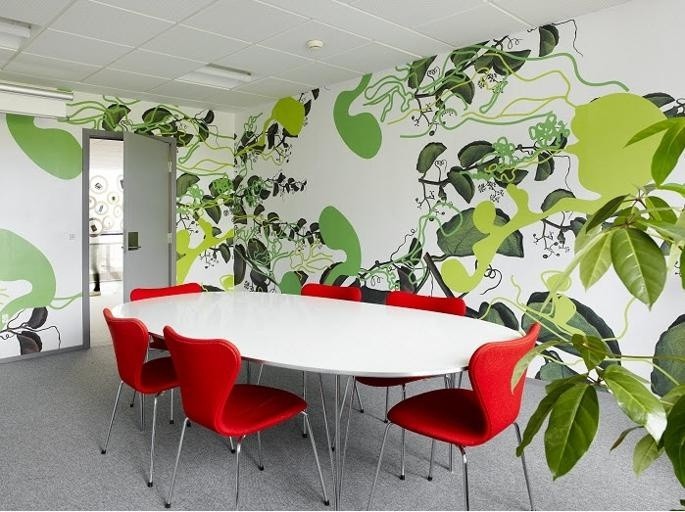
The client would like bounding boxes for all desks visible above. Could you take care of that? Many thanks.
[108,290,528,511]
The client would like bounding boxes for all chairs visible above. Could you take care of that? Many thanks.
[162,324,330,508]
[130,284,203,409]
[365,321,543,510]
[300,283,365,415]
[100,307,179,488]
[346,289,468,422]
